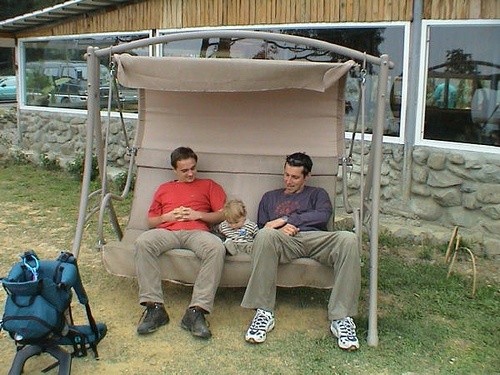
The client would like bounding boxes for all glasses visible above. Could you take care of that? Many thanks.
[287,155,311,172]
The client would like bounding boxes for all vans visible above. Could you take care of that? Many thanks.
[26,61,114,108]
[117,80,138,103]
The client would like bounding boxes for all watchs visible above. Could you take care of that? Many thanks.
[282,215,289,224]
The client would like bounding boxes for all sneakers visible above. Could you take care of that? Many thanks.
[137,302,170,334]
[182,307,212,338]
[244,308,275,343]
[330,316,359,350]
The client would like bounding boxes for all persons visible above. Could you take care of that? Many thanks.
[211,199,260,256]
[241,152,361,352]
[134,147,226,339]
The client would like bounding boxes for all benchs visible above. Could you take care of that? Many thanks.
[101,53,356,288]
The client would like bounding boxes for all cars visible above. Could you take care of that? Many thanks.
[1,75,17,102]
